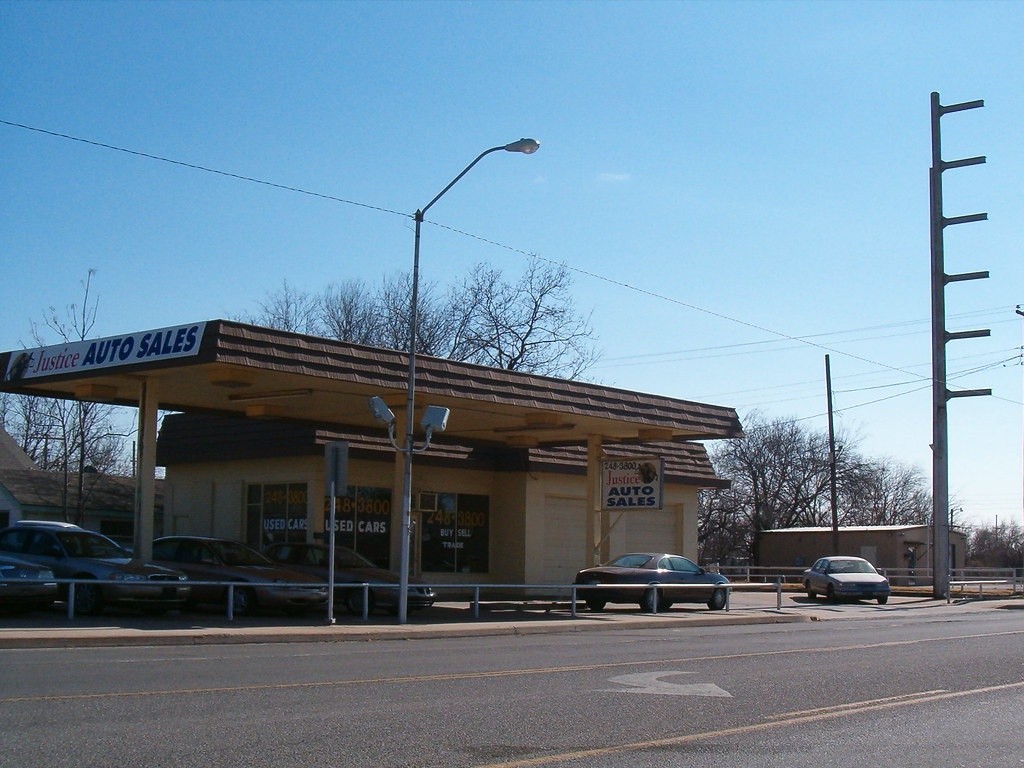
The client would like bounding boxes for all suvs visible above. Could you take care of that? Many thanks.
[1,521,192,617]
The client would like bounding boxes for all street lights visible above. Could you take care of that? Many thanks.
[367,139,541,622]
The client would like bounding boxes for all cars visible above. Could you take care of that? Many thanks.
[238,541,438,615]
[803,556,892,604]
[574,552,732,614]
[151,535,330,616]
[0,557,58,611]
